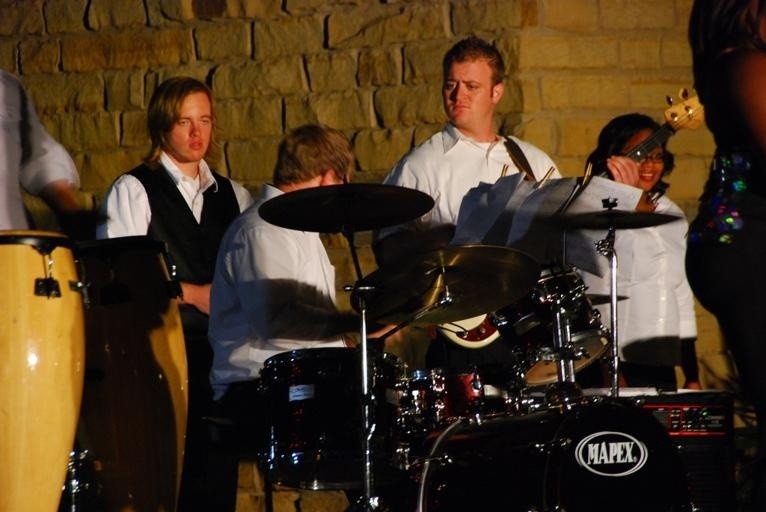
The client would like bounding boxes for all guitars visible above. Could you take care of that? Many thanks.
[595,89,704,212]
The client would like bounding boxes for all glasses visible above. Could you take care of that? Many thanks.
[640,153,664,163]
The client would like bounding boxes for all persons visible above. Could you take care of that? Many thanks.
[0,68,85,232]
[578,111,703,391]
[207,122,408,425]
[369,37,641,379]
[96,74,257,326]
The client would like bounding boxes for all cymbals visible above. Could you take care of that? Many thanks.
[553,209,683,232]
[588,292,632,305]
[351,243,541,323]
[258,183,435,233]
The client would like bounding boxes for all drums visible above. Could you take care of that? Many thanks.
[258,347,408,471]
[417,396,696,512]
[488,272,614,386]
[384,363,485,438]
[0,231,86,512]
[72,237,189,512]
